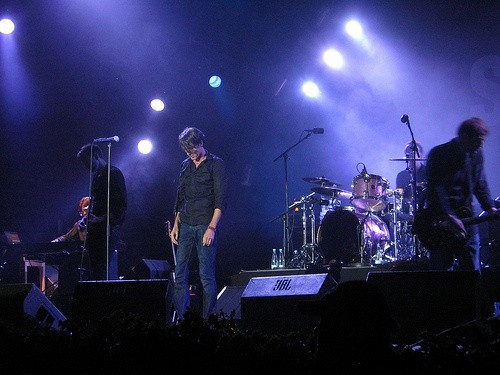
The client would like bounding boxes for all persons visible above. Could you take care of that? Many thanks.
[171,127,225,319]
[413,117,500,272]
[78,143,127,280]
[393,141,427,208]
[299,282,398,375]
[50,196,91,242]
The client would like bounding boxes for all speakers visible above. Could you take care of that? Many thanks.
[214,284,248,323]
[240,272,346,332]
[0,283,68,334]
[70,279,185,329]
[363,270,471,330]
[124,259,172,279]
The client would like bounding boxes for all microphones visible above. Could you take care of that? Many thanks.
[94,136,120,143]
[401,115,408,123]
[363,165,370,183]
[304,128,324,134]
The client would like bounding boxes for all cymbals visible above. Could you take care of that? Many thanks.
[302,176,343,187]
[310,186,352,200]
[389,157,425,161]
[409,182,428,187]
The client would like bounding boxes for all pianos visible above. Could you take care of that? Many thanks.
[0,240,89,257]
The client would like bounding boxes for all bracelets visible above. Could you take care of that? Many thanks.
[208,225,216,232]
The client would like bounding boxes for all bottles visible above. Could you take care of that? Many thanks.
[271,249,278,270]
[279,249,285,269]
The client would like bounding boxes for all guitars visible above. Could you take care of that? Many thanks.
[413,204,500,250]
[78,194,96,241]
[164,219,178,292]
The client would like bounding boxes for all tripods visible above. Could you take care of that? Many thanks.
[371,190,410,263]
[287,199,326,270]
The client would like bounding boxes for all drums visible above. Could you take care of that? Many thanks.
[350,174,390,211]
[315,211,391,265]
[380,198,412,222]
